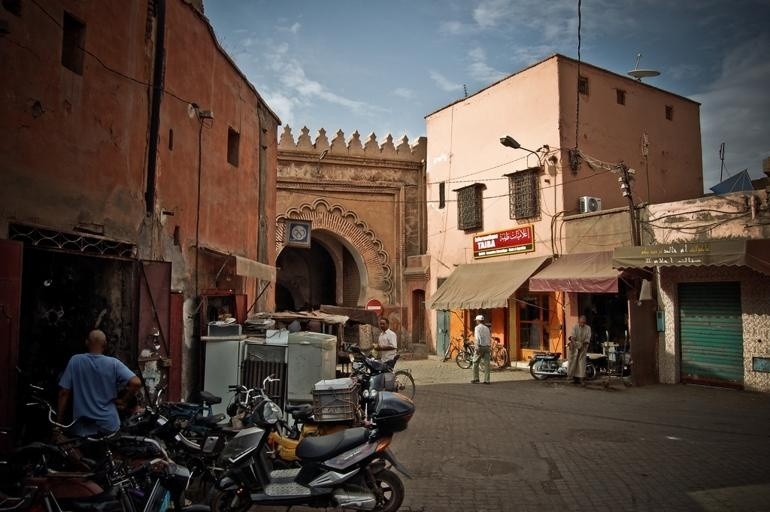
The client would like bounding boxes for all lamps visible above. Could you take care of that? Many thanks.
[499,135,545,172]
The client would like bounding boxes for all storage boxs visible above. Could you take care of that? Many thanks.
[315,377,353,390]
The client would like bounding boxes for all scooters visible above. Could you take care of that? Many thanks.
[209,387,416,512]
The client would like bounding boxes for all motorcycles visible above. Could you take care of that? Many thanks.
[0,346,416,512]
[528,335,597,381]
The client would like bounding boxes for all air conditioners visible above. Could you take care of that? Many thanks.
[579,196,602,214]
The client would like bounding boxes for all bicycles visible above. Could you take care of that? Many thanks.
[442,332,509,371]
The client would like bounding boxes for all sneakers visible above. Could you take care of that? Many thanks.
[470,379,479,383]
[484,382,490,384]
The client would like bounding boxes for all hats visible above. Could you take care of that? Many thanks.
[474,315,484,321]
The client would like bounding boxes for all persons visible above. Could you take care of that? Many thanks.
[373,317,398,373]
[469,315,491,384]
[567,315,591,387]
[57,329,142,461]
